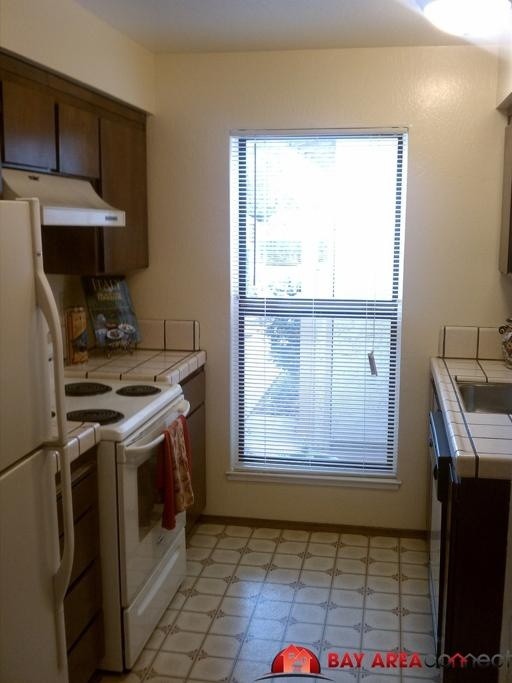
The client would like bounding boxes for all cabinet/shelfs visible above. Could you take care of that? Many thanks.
[1,48,149,277]
[428,381,512,683]
[55,443,106,683]
[179,365,206,545]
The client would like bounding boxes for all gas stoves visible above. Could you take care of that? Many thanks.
[64,377,181,440]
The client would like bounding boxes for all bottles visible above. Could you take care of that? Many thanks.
[497,319,512,369]
[66,306,89,367]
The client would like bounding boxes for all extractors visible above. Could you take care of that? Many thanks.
[3,169,131,229]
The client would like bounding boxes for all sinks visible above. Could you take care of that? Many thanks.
[456,381,512,414]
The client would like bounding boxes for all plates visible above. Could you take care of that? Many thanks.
[106,323,136,339]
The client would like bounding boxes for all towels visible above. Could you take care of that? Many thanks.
[156,415,194,530]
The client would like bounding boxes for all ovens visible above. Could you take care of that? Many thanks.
[115,389,193,672]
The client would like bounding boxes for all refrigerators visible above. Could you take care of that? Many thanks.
[0,198,74,683]
